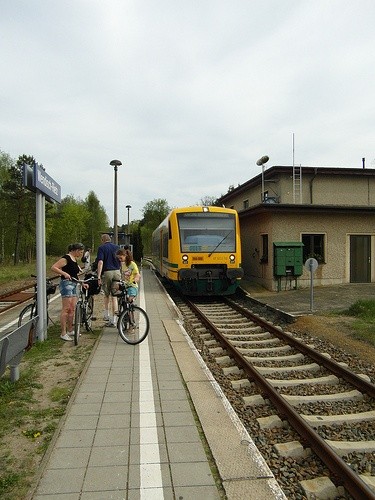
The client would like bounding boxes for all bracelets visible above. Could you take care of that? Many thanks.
[98,279,101,280]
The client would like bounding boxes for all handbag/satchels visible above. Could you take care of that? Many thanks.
[81,257,86,262]
[82,273,100,295]
[35,284,55,295]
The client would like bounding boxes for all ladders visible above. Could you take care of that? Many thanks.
[293,133,302,204]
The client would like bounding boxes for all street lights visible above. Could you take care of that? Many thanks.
[109,159,122,245]
[126,205,132,228]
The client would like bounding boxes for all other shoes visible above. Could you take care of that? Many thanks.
[103,316,109,321]
[105,322,116,327]
[60,334,72,341]
[67,330,75,335]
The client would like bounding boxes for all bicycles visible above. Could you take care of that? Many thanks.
[17,274,62,328]
[111,278,150,345]
[60,273,102,345]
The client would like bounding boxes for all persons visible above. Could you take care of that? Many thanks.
[114,248,141,334]
[51,242,89,341]
[97,234,121,328]
[84,248,91,269]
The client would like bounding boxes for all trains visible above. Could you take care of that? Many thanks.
[151,205,244,299]
